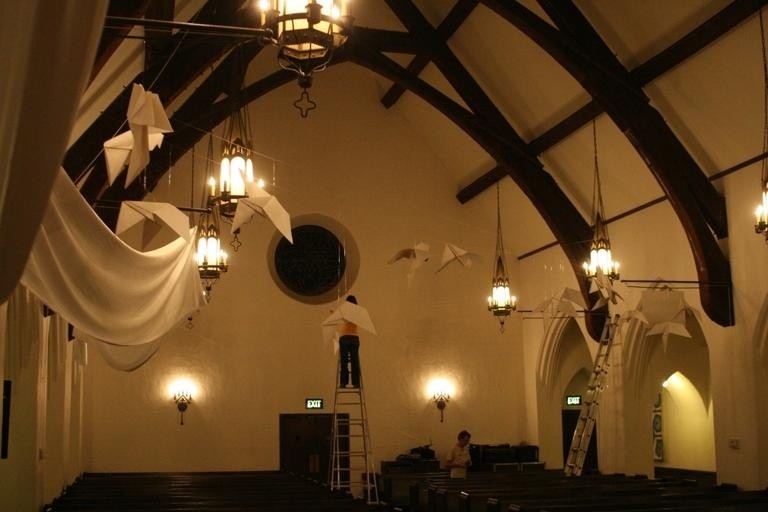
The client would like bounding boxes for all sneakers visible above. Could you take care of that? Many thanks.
[340,384,360,388]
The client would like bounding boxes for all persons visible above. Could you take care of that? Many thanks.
[330,295,362,388]
[445,429,473,479]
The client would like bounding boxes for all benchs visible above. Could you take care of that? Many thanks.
[39,463,767,512]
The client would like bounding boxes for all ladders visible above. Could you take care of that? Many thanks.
[331,351,378,504]
[563,314,621,476]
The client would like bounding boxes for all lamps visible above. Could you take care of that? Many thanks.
[581,116,622,286]
[486,158,516,329]
[749,9,768,244]
[194,44,259,304]
[256,1,353,120]
